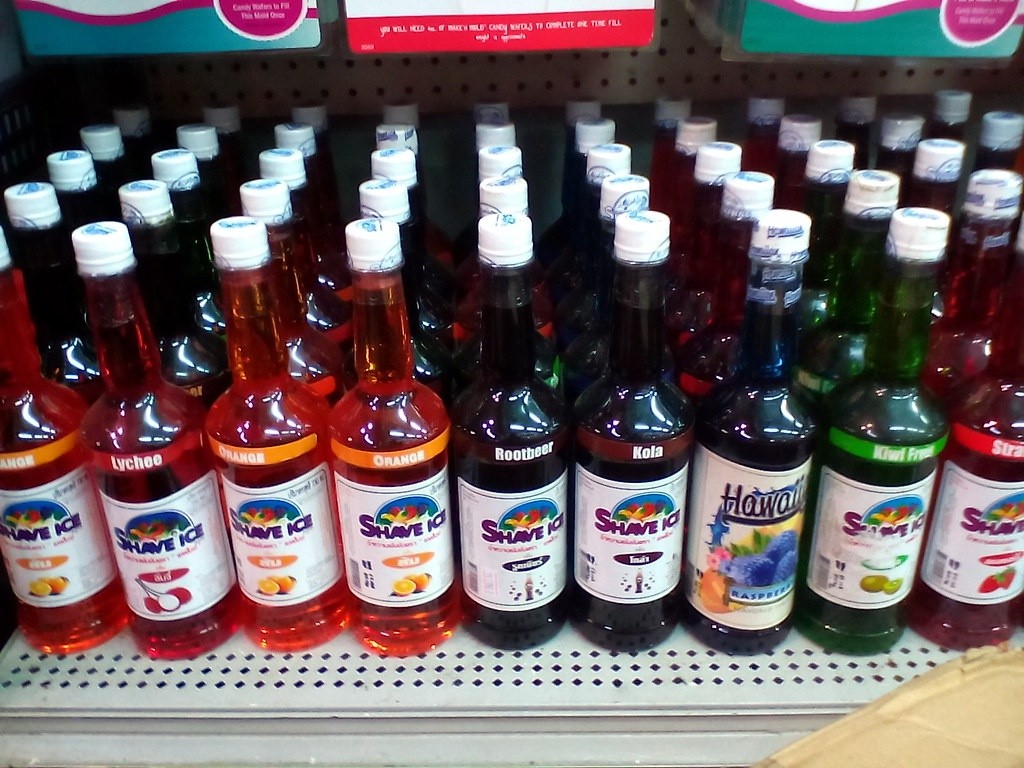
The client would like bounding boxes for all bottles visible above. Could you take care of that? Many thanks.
[0,91,1024,664]
